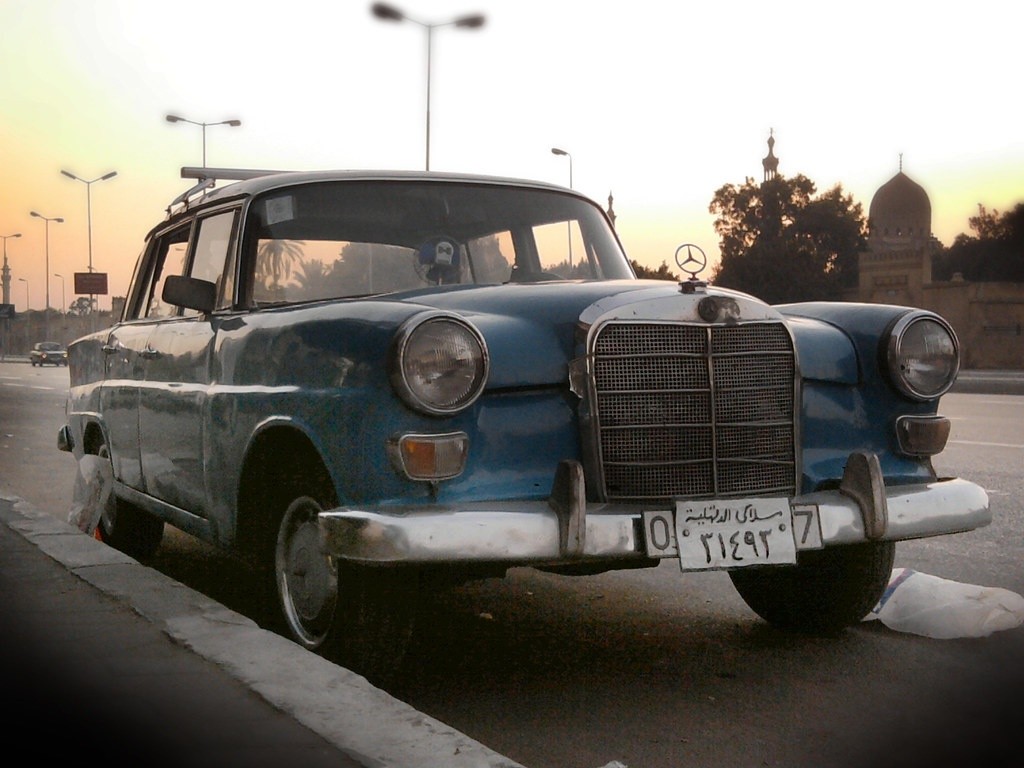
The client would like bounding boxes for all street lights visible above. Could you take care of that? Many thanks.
[166,114,242,195]
[0,232,21,305]
[87,266,100,314]
[18,278,29,314]
[28,210,65,341]
[54,273,65,320]
[60,169,118,335]
[367,2,490,177]
[549,147,575,277]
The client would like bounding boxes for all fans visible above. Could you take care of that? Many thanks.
[412,232,467,287]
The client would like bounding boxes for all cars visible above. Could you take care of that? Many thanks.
[30,341,68,367]
[58,164,992,670]
